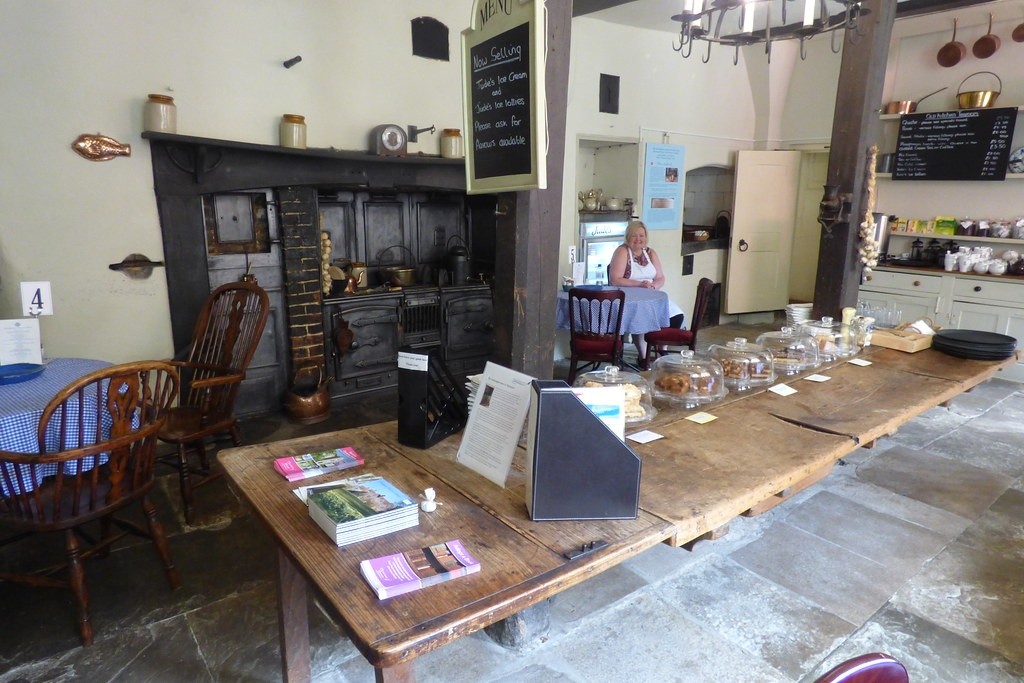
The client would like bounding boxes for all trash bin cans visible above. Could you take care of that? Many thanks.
[786,304,812,326]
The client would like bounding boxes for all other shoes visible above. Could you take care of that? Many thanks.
[636,356,652,371]
[658,348,668,356]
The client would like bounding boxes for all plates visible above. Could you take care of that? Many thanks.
[785,302,812,329]
[931,328,1017,360]
[624,341,860,430]
[575,284,603,291]
[886,257,908,260]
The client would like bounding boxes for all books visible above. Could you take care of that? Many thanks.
[306,476,419,547]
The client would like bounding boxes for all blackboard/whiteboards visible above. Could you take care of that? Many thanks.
[459,0,548,195]
[891,106,1019,181]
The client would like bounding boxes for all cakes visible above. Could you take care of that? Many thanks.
[772,344,808,365]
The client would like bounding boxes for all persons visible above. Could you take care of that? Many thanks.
[609,221,683,371]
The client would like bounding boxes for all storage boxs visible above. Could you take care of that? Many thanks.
[891,215,957,235]
[870,328,933,353]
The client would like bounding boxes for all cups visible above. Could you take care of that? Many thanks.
[849,299,902,347]
[943,245,991,273]
[902,252,909,258]
[842,307,857,325]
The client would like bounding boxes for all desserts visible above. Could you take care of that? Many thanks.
[581,380,645,416]
[715,359,769,378]
[653,371,718,393]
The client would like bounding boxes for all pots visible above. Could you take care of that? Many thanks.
[379,245,419,287]
[885,12,1024,114]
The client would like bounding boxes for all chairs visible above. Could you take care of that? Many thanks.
[0,282,269,647]
[644,278,714,372]
[568,288,625,386]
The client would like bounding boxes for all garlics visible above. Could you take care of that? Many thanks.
[321,233,331,293]
[858,222,879,269]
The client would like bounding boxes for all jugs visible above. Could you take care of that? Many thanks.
[911,238,959,265]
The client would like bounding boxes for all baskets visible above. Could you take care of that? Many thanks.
[694,232,710,241]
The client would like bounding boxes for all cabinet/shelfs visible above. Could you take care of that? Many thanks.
[875,105,1024,244]
[855,266,1024,384]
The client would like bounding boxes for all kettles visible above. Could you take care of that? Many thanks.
[284,360,334,425]
[332,234,471,298]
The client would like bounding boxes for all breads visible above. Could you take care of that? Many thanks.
[811,334,836,351]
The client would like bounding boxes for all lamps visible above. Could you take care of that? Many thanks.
[671,0,872,65]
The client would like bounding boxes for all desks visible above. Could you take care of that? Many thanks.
[0,358,140,498]
[557,284,669,372]
[213,342,1024,683]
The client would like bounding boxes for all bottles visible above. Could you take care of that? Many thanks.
[957,216,1024,239]
[144,94,177,134]
[440,128,463,158]
[280,113,306,149]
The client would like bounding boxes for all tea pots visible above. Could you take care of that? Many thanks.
[578,188,624,211]
[973,250,1024,276]
